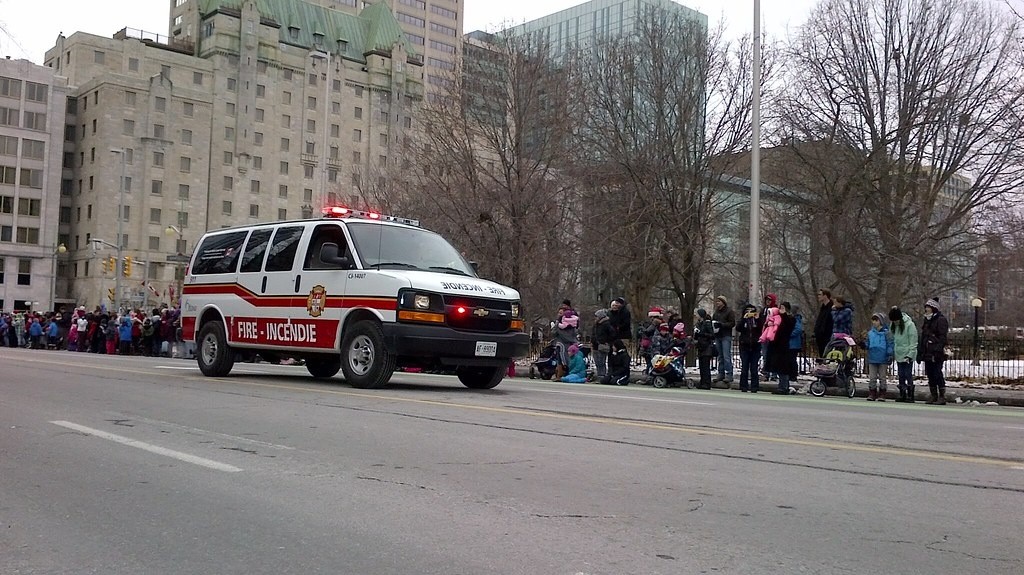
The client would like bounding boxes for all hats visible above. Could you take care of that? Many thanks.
[613,339,624,350]
[764,294,799,316]
[717,296,727,306]
[659,320,684,356]
[567,343,578,354]
[595,296,625,319]
[924,296,940,312]
[559,300,573,318]
[698,309,706,318]
[648,307,664,317]
[873,305,903,325]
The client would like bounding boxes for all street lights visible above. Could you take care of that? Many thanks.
[166,195,184,308]
[89,146,126,313]
[972,298,982,367]
[49,243,66,312]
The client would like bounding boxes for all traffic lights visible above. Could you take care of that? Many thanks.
[107,288,116,302]
[124,257,131,277]
[109,258,114,271]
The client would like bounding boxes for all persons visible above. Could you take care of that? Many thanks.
[736,304,763,393]
[916,296,948,404]
[815,288,835,358]
[832,294,854,335]
[888,305,919,403]
[551,296,689,387]
[0,304,180,357]
[692,309,714,389]
[711,295,735,382]
[789,304,802,385]
[759,293,777,382]
[764,301,796,395]
[759,307,781,342]
[860,312,894,402]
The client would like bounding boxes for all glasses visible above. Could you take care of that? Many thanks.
[765,296,772,301]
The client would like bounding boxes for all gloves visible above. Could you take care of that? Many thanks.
[916,354,923,364]
[857,339,866,348]
[887,356,893,365]
[904,356,912,362]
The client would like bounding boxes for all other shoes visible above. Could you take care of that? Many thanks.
[741,375,797,395]
[696,382,711,390]
[723,374,733,382]
[713,374,725,382]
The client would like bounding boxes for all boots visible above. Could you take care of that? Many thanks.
[878,386,887,401]
[895,384,907,401]
[905,385,915,403]
[867,386,878,401]
[925,387,938,403]
[932,389,946,405]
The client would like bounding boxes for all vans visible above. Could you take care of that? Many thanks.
[172,206,530,389]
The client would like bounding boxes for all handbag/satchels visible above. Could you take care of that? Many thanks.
[598,344,610,353]
[553,365,569,382]
[640,337,651,348]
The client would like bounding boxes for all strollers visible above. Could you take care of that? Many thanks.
[809,334,859,398]
[649,330,695,389]
[531,345,561,380]
